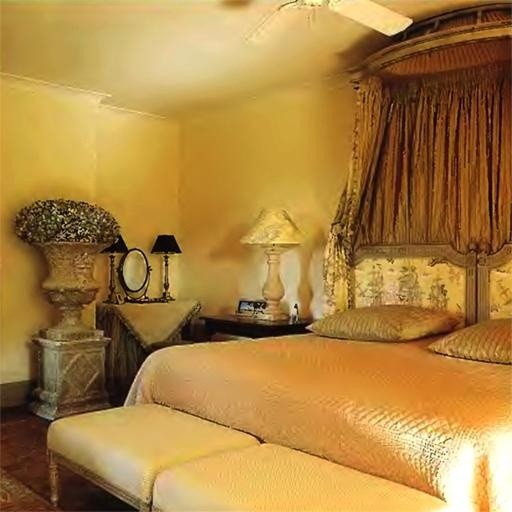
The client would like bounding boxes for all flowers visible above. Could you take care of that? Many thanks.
[11,196,122,244]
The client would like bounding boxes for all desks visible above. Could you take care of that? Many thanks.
[96,299,202,407]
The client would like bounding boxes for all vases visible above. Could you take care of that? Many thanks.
[28,246,114,422]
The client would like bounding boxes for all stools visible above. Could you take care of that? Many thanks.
[152,441,447,510]
[46,403,260,511]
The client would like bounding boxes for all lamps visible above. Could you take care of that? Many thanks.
[239,207,306,323]
[97,234,129,303]
[151,233,183,301]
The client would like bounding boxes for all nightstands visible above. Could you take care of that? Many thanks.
[189,313,313,342]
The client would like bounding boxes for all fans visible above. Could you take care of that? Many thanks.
[246,0,415,44]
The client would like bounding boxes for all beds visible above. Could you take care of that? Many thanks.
[143,236,511,512]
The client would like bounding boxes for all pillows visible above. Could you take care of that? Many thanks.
[305,303,512,366]
